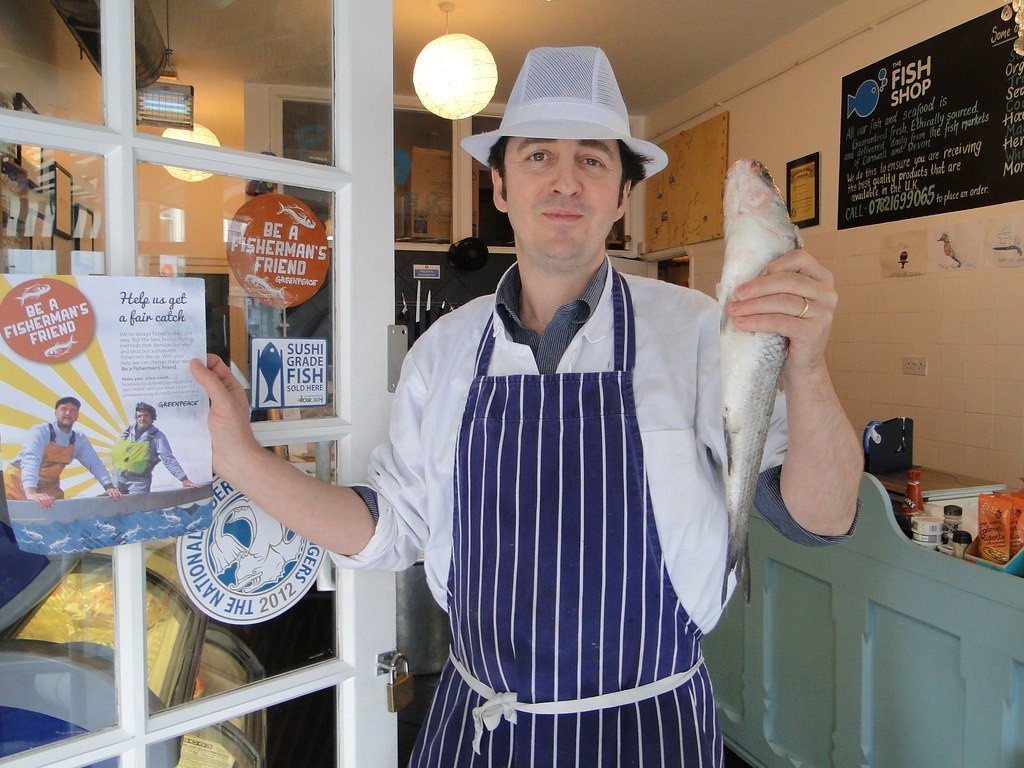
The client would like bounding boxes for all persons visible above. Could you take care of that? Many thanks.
[112,401,204,496]
[3,396,123,511]
[190,42,866,768]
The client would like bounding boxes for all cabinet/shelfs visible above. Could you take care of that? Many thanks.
[240,80,646,261]
[701,470,1022,767]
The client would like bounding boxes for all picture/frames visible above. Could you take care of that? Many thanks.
[786,151,819,229]
[50,161,75,240]
[15,92,43,194]
[75,203,94,253]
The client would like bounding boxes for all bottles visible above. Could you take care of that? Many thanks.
[900,468,925,540]
[941,505,962,546]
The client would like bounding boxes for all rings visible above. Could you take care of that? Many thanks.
[796,297,809,318]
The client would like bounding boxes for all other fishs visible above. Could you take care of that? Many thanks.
[713,156,808,610]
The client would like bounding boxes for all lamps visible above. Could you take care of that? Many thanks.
[135,0,195,132]
[412,1,498,121]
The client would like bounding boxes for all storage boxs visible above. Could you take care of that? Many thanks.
[963,536,1024,579]
[394,146,479,241]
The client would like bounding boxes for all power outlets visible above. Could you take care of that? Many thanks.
[900,357,929,375]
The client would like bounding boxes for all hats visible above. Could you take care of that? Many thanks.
[57,397,81,408]
[137,403,157,419]
[461,45,669,182]
